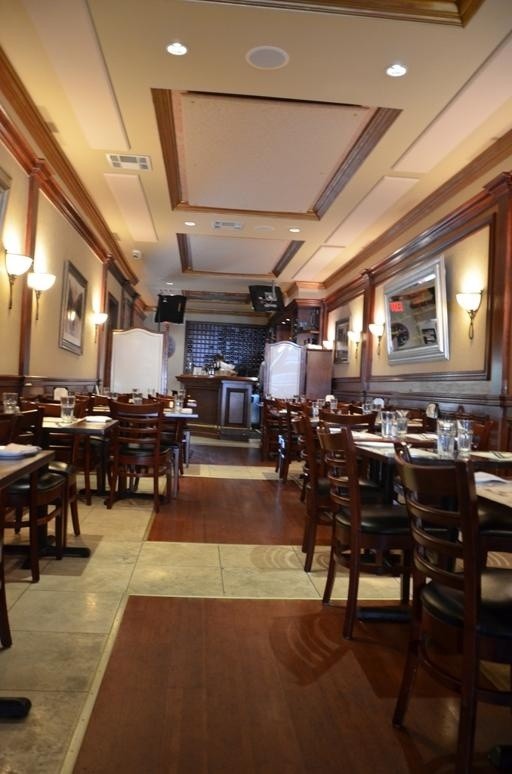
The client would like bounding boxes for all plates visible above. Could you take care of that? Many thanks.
[1,445,39,460]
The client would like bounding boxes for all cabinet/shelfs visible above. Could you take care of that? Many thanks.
[262,298,324,344]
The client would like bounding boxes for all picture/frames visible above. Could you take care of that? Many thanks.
[383,255,448,366]
[59,259,88,357]
[334,317,351,365]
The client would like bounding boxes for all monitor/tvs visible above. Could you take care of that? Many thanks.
[155,295,186,323]
[249,285,284,312]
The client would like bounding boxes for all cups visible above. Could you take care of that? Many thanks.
[396,408,409,433]
[100,386,186,413]
[457,419,473,452]
[4,391,19,412]
[380,410,395,437]
[60,395,76,422]
[436,420,455,454]
[264,393,374,420]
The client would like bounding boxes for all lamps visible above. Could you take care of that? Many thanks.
[323,321,383,362]
[30,270,55,325]
[2,252,31,311]
[456,290,481,345]
[92,307,111,348]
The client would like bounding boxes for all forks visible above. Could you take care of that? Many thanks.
[489,450,512,460]
[417,432,433,439]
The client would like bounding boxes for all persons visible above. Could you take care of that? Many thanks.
[207,352,236,373]
